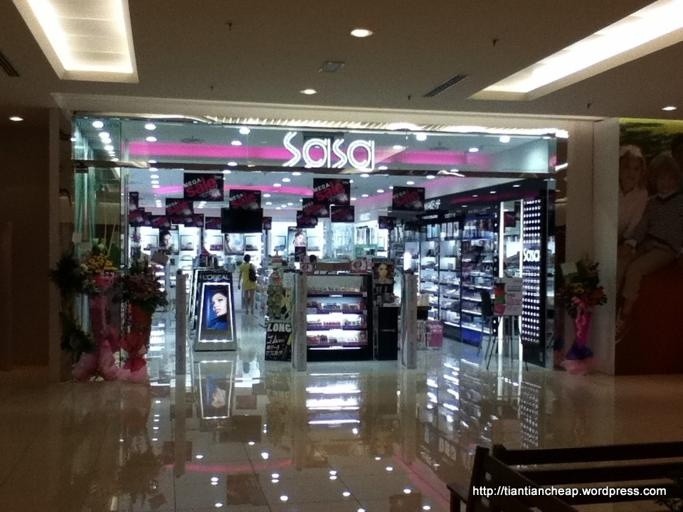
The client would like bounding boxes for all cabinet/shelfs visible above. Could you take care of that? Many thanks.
[295,270,372,361]
[389,203,498,348]
[254,259,275,328]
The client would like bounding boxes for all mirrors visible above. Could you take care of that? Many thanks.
[500,199,522,360]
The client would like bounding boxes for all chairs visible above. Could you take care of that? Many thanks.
[475,287,512,360]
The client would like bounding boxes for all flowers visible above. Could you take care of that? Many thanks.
[111,247,168,308]
[558,252,608,319]
[75,238,116,275]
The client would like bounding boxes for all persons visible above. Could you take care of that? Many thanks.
[310,254,317,264]
[237,255,257,314]
[159,231,177,251]
[293,231,305,245]
[225,233,243,254]
[370,249,376,255]
[615,132,683,345]
[375,264,393,283]
[210,291,228,329]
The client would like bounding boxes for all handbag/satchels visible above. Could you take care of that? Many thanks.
[248,268,256,281]
